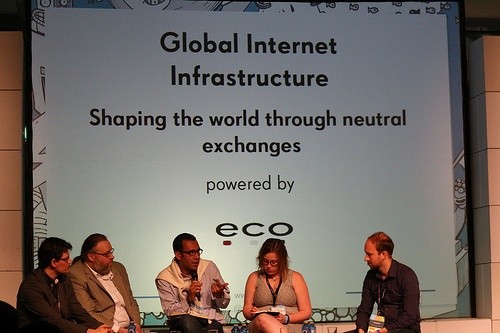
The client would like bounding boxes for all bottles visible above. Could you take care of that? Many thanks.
[128,321,136,333]
[231,323,248,333]
[302,321,316,333]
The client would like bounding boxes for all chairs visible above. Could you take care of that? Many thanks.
[0,301,17,333]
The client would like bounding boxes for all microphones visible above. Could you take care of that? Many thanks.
[191,270,201,301]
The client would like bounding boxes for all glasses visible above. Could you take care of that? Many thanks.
[182,249,203,256]
[262,258,278,266]
[92,248,114,258]
[59,255,70,263]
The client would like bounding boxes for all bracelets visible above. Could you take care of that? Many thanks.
[377,330,380,333]
[283,314,290,325]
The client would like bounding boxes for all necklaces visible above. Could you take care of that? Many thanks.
[270,275,281,282]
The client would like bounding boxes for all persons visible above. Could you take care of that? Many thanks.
[154,233,231,333]
[243,238,312,333]
[17,237,114,333]
[64,233,143,333]
[342,232,422,333]
